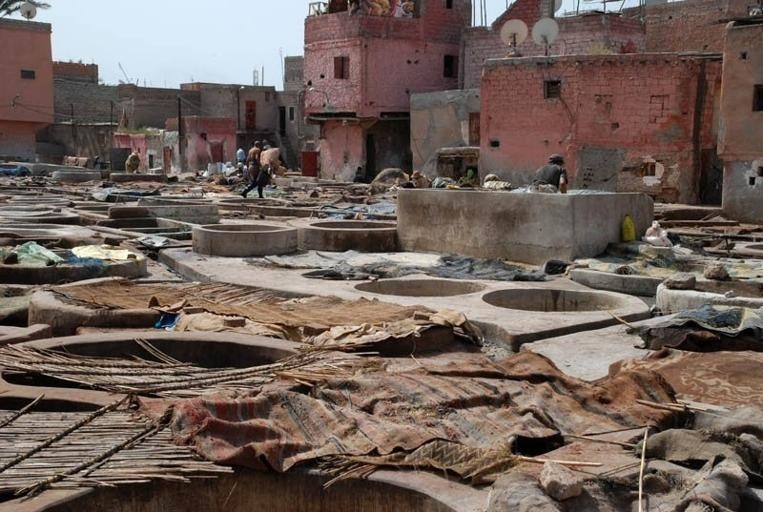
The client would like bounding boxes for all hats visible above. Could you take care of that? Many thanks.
[132,151,139,156]
[548,153,563,163]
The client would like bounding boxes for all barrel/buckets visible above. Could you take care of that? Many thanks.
[622,213,635,242]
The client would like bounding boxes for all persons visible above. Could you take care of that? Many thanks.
[528,152,568,195]
[233,144,246,176]
[241,143,282,199]
[246,140,261,182]
[262,139,270,151]
[123,151,141,174]
